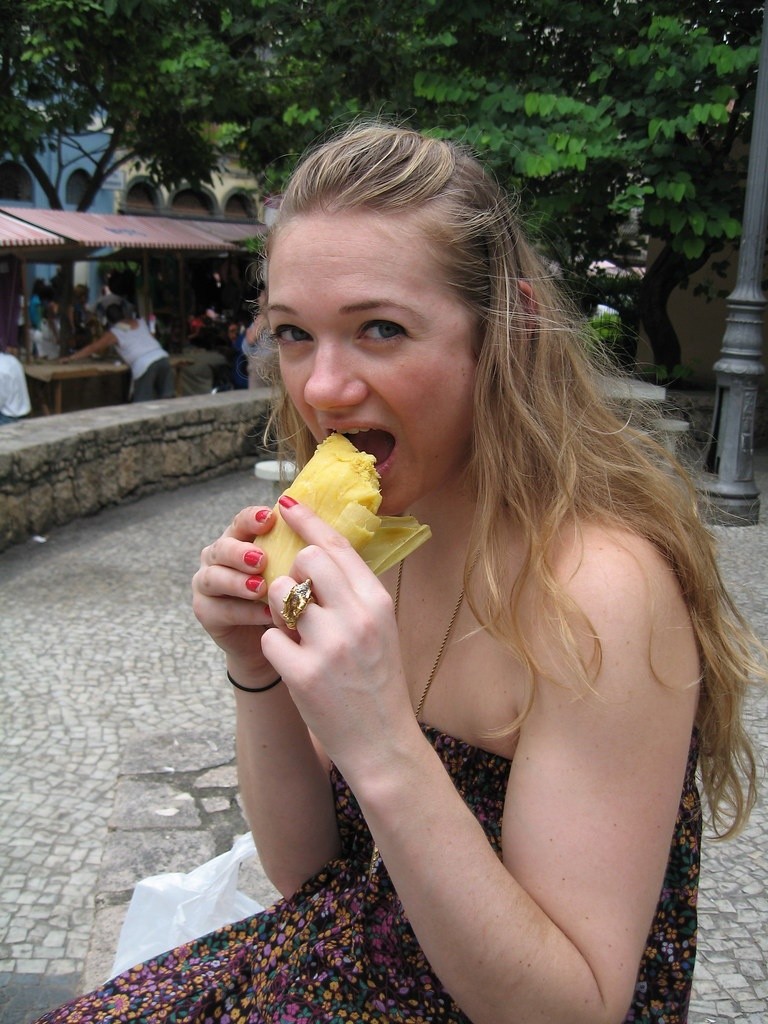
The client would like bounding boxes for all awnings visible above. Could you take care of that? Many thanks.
[0,207,270,363]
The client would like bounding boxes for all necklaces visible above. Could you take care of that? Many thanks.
[370,540,481,873]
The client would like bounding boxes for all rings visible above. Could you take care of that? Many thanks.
[280,579,316,630]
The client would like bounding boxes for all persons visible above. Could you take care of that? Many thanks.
[0,268,277,428]
[32,125,768,1024]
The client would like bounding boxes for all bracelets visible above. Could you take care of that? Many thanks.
[226,670,282,693]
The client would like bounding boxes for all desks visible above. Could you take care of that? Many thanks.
[24,349,223,421]
